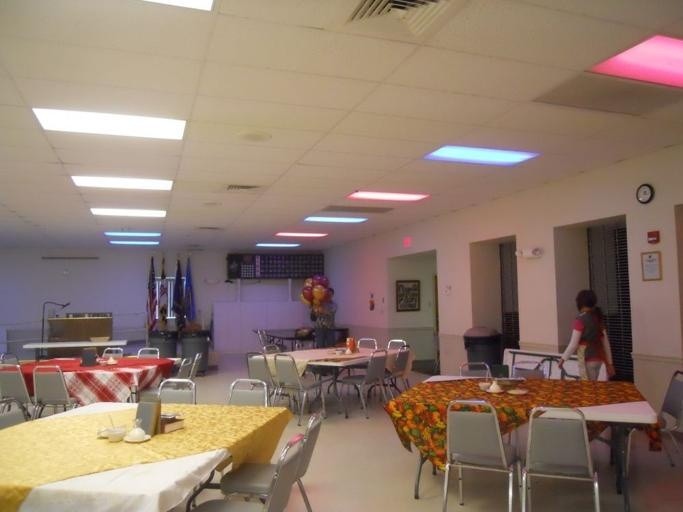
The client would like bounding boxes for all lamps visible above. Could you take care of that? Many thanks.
[109,241,159,245]
[104,231,161,236]
[71,176,174,191]
[586,35,682,87]
[345,190,432,203]
[31,110,187,139]
[256,243,301,247]
[148,0,216,11]
[275,232,328,238]
[89,207,167,218]
[424,146,541,166]
[304,217,369,223]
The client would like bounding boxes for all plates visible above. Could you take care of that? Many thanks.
[107,361,117,365]
[507,390,528,394]
[486,390,505,393]
[123,435,152,443]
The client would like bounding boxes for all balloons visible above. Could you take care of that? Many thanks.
[300,276,334,314]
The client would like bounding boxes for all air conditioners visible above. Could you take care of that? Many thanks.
[346,0,444,35]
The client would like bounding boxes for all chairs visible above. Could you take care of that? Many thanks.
[340,351,387,419]
[32,366,79,419]
[460,362,490,377]
[137,348,159,358]
[102,348,123,358]
[227,378,267,406]
[346,340,378,395]
[157,378,196,404]
[157,359,192,389]
[275,354,328,426]
[521,406,601,511]
[265,345,282,353]
[0,365,32,420]
[189,354,202,379]
[186,434,304,512]
[381,340,413,392]
[374,345,410,400]
[195,411,321,511]
[444,400,521,512]
[512,361,544,377]
[246,353,276,406]
[625,370,682,478]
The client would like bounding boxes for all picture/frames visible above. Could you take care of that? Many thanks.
[396,280,419,311]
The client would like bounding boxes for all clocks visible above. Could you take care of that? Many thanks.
[636,184,653,203]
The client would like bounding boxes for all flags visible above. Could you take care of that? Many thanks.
[172,260,185,339]
[145,257,158,337]
[159,259,168,334]
[185,258,196,323]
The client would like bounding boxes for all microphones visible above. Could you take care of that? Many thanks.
[62,302,71,310]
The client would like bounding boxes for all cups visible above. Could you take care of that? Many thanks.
[108,357,114,364]
[489,381,501,392]
[345,349,352,354]
[130,427,146,440]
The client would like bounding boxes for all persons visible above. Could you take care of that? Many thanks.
[311,288,338,376]
[559,288,615,382]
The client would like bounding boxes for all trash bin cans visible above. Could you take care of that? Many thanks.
[181,330,210,377]
[464,326,504,371]
[148,330,178,358]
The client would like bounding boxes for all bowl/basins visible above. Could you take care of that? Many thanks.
[99,361,107,366]
[479,383,490,390]
[107,428,125,442]
[336,351,342,356]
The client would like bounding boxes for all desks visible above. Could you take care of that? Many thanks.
[0,356,179,417]
[384,376,657,512]
[23,340,128,350]
[0,402,290,512]
[250,330,349,339]
